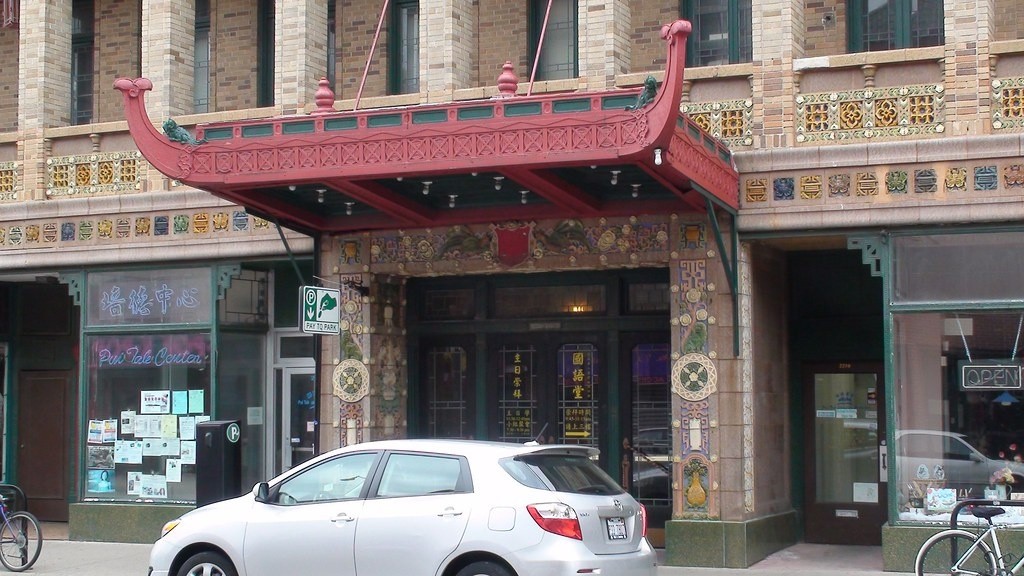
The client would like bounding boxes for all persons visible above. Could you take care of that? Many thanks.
[98,470,111,491]
[323,459,372,499]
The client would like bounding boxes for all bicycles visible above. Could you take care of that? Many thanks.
[913,505,1024,576]
[0,491,43,572]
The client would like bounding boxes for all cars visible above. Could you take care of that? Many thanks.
[817,426,1024,514]
[149,439,658,576]
